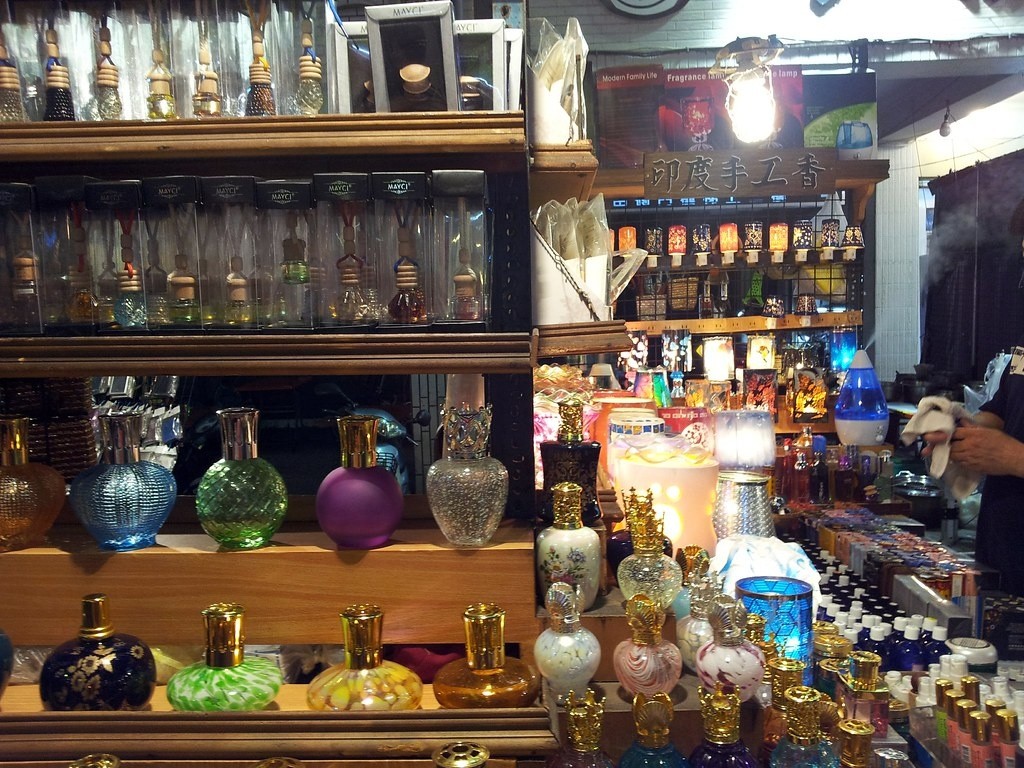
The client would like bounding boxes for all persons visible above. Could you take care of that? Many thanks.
[920,337,1024,595]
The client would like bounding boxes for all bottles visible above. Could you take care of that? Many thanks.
[884,652,1024,768]
[777,531,950,670]
[782,427,892,504]
[981,597,1024,660]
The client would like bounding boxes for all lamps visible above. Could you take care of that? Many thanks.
[707,35,784,147]
[602,218,865,558]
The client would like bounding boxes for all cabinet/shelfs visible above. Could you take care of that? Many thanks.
[0,107,766,768]
